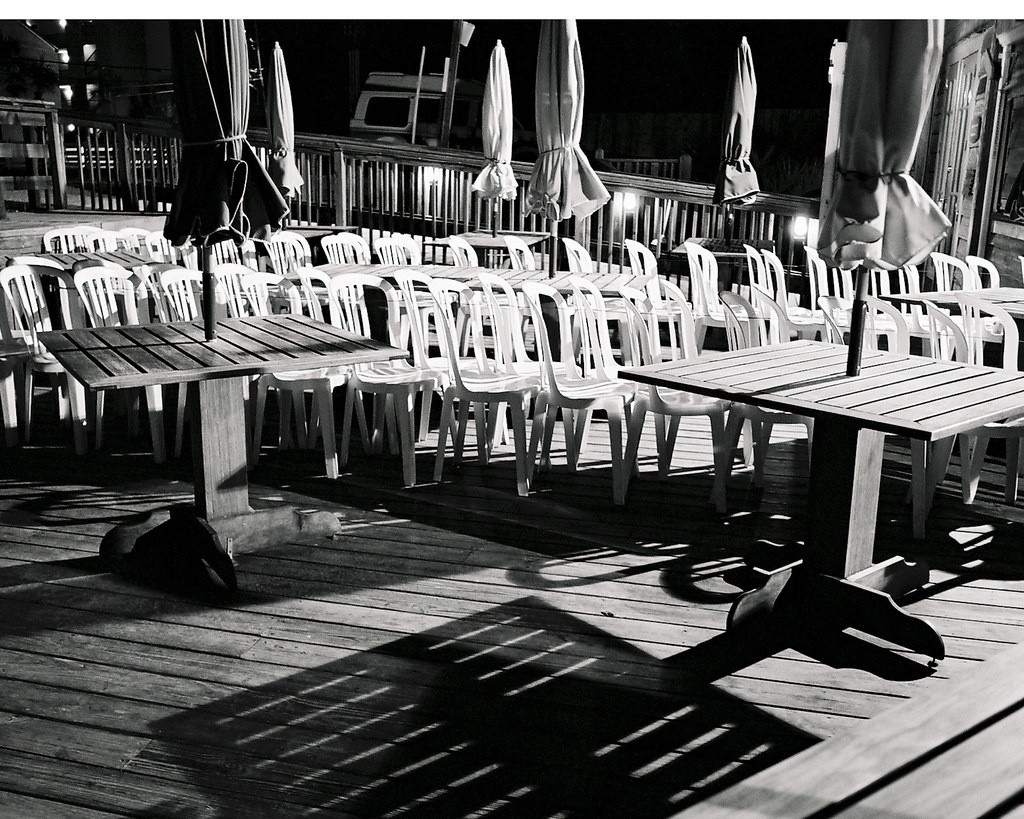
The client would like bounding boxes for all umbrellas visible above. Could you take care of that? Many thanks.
[162,19,288,252]
[709,32,762,212]
[266,41,304,200]
[815,19,950,278]
[520,18,610,225]
[471,37,520,204]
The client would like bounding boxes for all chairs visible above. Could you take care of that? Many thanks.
[0,222,1024,544]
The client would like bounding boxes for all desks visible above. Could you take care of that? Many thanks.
[30,312,411,601]
[617,337,1024,662]
